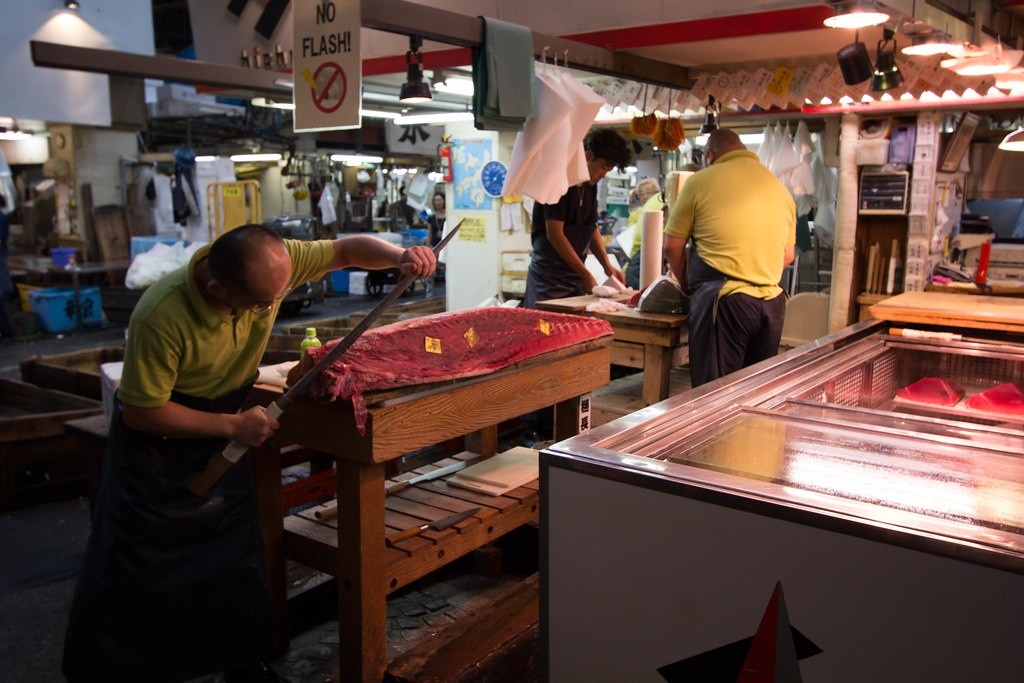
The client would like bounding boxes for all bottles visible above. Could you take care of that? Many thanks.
[299,328,321,359]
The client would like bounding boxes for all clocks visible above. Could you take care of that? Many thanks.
[480,161,508,197]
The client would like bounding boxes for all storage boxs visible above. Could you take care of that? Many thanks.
[16,281,46,313]
[500,271,529,293]
[501,249,532,273]
[26,284,104,333]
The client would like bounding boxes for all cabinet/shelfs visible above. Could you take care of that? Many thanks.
[245,328,612,683]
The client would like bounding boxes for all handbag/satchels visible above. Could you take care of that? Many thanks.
[437,231,443,238]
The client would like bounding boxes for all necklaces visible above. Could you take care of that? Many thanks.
[576,181,586,207]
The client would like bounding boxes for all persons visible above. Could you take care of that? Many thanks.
[392,187,418,227]
[307,182,333,240]
[424,191,445,300]
[621,178,664,263]
[628,190,642,228]
[523,127,626,311]
[661,129,797,389]
[59,224,439,682]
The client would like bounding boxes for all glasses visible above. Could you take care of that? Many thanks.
[637,190,659,198]
[224,278,296,315]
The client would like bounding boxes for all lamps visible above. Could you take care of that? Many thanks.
[822,0,889,29]
[868,24,906,93]
[393,109,477,126]
[937,112,960,136]
[694,94,723,146]
[398,36,433,104]
[429,67,474,99]
[900,29,964,57]
[836,28,874,87]
[249,92,407,120]
[997,113,1024,153]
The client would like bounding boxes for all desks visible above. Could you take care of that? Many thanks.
[6,252,133,333]
[533,286,690,408]
